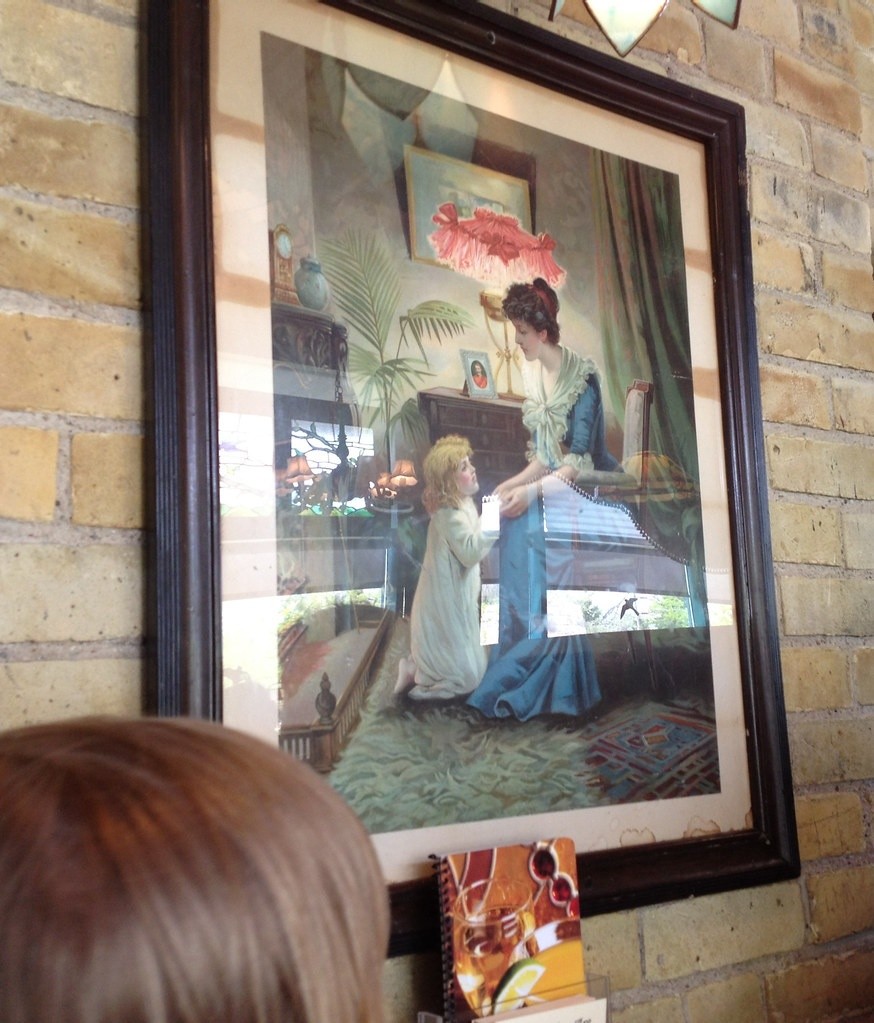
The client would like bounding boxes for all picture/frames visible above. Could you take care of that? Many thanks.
[134,2,802,957]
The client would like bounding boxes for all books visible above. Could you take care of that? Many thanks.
[427,837,608,1023]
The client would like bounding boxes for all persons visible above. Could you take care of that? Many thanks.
[0,712,395,1023]
